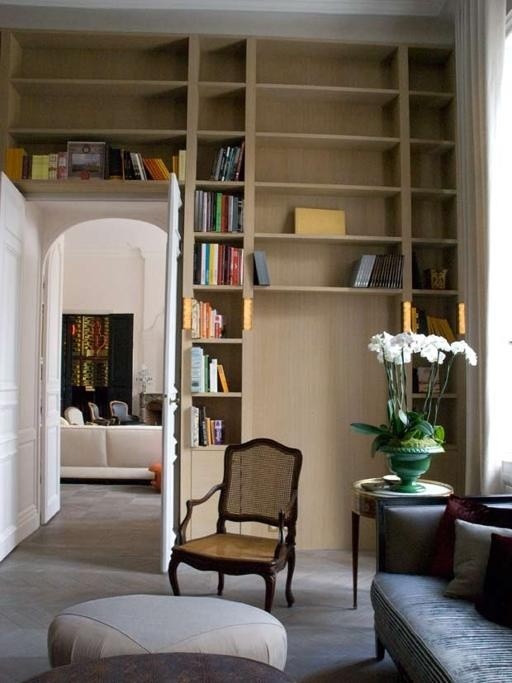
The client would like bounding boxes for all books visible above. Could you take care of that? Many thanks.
[5,137,186,185]
[251,251,271,285]
[353,254,406,289]
[409,307,457,394]
[191,143,244,445]
[294,208,345,238]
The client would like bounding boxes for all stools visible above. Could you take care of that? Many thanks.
[22,592,292,683]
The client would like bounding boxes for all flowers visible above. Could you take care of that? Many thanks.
[349,330,477,461]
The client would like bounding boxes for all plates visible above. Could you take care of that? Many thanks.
[361,483,384,491]
[383,475,399,482]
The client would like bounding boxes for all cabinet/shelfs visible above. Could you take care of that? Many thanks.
[0,25,467,454]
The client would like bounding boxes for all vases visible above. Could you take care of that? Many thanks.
[378,447,445,493]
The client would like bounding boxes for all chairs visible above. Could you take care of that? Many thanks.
[168,436,303,613]
[65,400,141,425]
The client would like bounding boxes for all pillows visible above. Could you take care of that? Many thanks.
[421,492,512,629]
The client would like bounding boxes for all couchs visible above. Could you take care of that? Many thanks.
[60,424,163,485]
[369,492,512,682]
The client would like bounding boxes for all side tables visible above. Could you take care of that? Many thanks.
[349,477,454,610]
